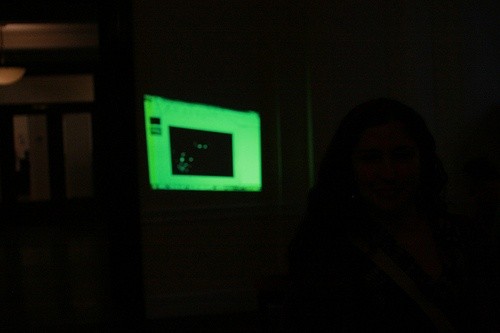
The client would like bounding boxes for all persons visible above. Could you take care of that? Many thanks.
[287,98,500,333]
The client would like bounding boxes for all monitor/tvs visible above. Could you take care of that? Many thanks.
[142,92,264,193]
